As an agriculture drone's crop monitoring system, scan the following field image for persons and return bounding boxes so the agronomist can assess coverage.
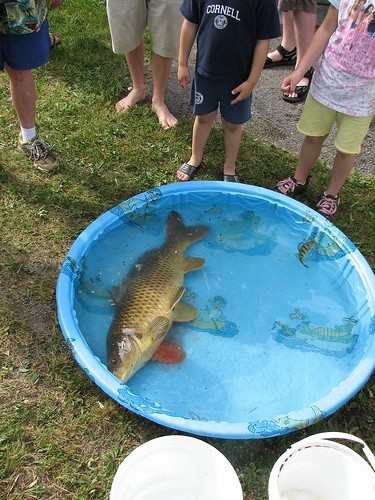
[176,0,281,185]
[270,0,375,219]
[0,0,66,173]
[262,1,319,102]
[106,0,185,131]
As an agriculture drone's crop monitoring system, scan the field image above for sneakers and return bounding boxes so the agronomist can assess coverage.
[273,175,312,197]
[315,191,340,220]
[18,130,59,174]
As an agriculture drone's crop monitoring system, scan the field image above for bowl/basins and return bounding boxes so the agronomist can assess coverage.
[56,180,375,440]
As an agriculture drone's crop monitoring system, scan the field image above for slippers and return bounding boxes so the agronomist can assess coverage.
[222,162,239,183]
[176,157,207,182]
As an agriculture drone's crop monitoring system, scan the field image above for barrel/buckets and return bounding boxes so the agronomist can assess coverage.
[267,431,375,500]
[108,435,243,500]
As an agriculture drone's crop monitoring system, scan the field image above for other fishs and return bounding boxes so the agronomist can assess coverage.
[108,210,211,383]
[298,240,316,268]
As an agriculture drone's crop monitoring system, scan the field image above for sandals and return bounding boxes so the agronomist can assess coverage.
[281,66,315,102]
[263,44,297,69]
